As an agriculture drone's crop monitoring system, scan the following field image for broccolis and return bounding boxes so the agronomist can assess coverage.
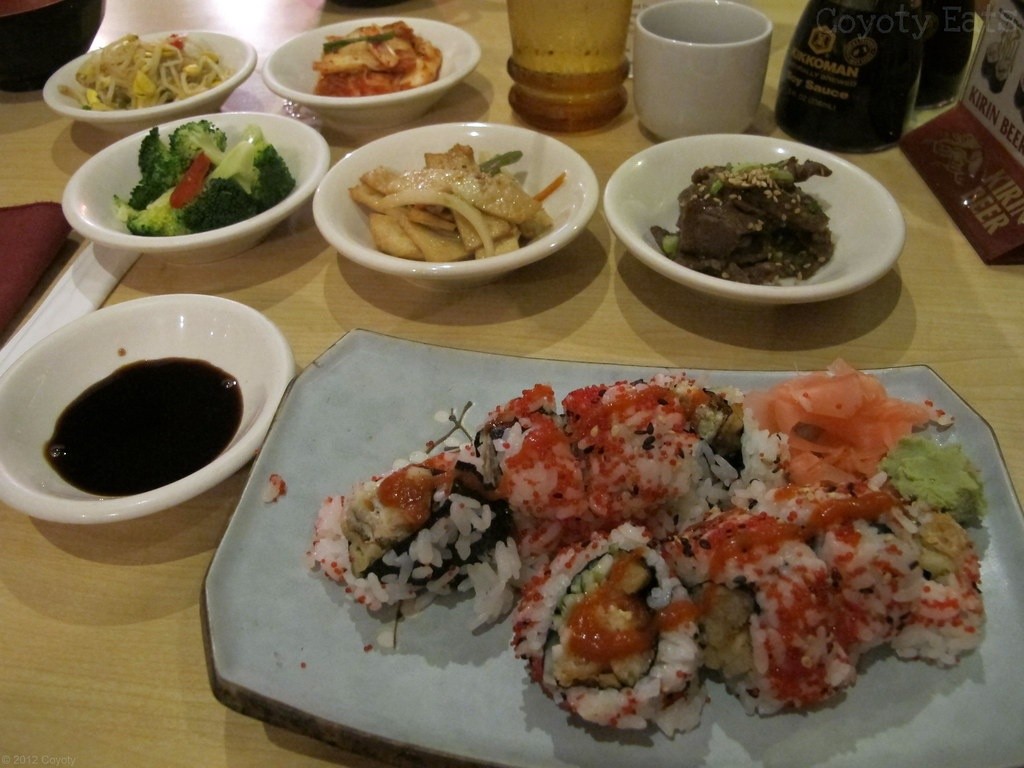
[114,120,294,237]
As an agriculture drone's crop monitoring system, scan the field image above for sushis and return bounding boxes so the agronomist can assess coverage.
[313,371,985,733]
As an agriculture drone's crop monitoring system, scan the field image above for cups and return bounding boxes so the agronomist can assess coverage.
[633,1,773,143]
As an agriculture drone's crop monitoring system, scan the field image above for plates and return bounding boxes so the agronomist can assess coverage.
[604,132,905,304]
[199,328,1024,768]
[42,30,258,136]
[312,121,600,286]
[0,293,301,522]
[260,15,483,128]
[60,111,332,266]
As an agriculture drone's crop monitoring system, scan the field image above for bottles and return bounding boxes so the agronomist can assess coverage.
[776,0,934,154]
[915,0,977,108]
